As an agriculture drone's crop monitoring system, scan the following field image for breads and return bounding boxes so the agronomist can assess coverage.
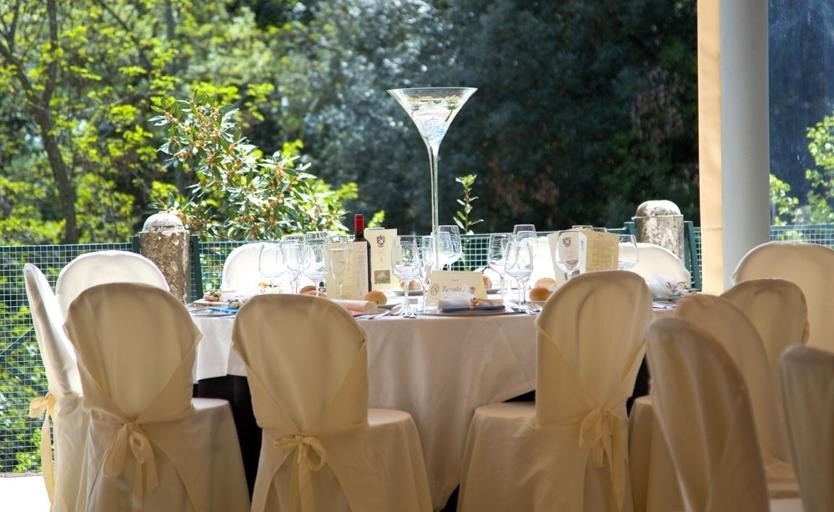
[299,286,316,293]
[408,280,422,291]
[307,290,325,297]
[366,290,388,304]
[400,281,403,286]
[530,287,549,300]
[537,278,556,287]
[481,274,493,289]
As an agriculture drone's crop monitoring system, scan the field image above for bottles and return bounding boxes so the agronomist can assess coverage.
[353,212,373,292]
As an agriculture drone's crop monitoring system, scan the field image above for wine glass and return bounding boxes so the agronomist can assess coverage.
[385,83,480,269]
[278,230,352,315]
[389,223,463,317]
[488,222,641,312]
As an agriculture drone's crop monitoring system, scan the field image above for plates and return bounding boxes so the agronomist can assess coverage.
[190,309,237,318]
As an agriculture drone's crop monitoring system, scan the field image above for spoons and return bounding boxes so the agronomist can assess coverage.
[366,302,404,321]
